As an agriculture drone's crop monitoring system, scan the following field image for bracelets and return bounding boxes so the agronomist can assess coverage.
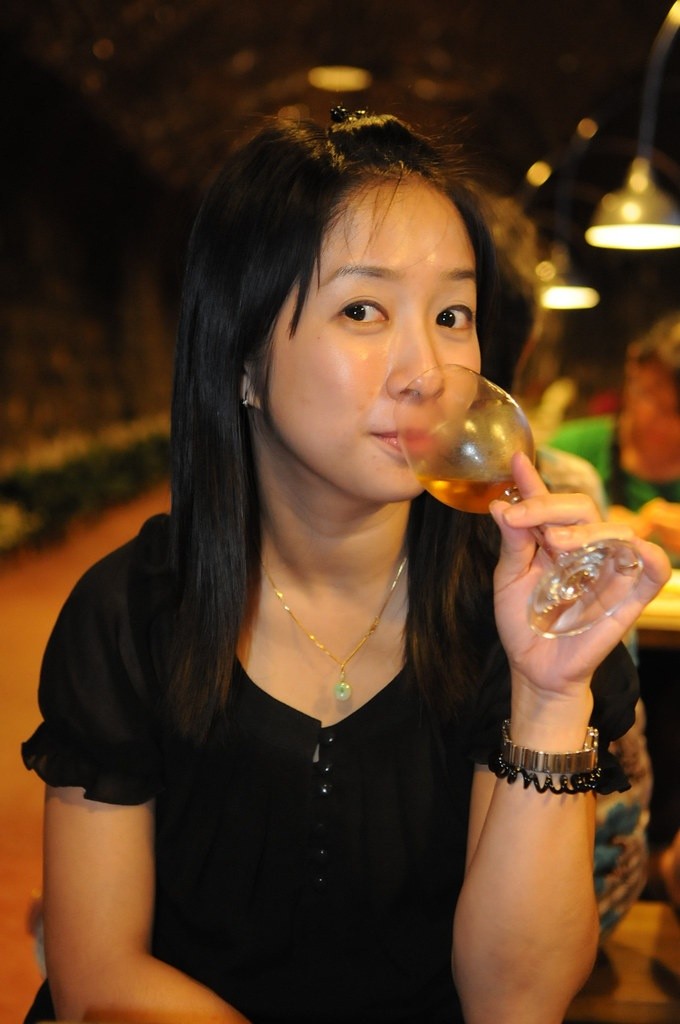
[487,758,604,794]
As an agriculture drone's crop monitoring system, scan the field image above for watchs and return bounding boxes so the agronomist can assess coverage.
[499,720,600,776]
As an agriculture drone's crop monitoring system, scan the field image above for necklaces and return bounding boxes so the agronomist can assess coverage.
[247,542,411,703]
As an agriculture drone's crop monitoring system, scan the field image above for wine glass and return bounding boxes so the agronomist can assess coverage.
[392,365,640,641]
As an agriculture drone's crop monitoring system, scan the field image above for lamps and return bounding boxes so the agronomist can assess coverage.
[586,158,679,249]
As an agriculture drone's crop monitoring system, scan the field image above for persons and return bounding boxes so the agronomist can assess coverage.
[18,100,672,1024]
[540,311,679,910]
